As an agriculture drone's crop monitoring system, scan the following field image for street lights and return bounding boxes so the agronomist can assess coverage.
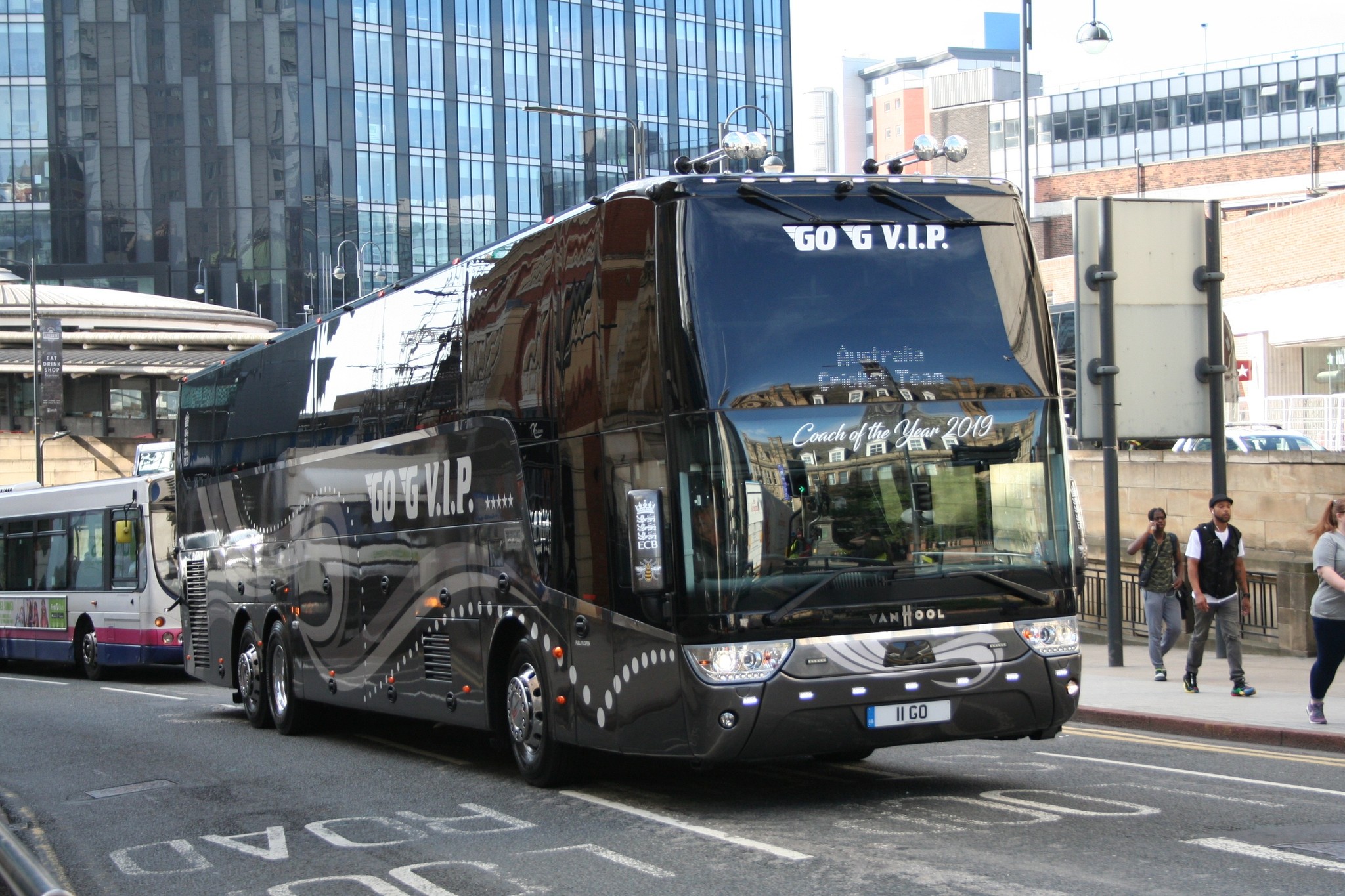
[718,105,787,175]
[1019,0,1113,222]
[36,429,72,486]
[193,258,208,304]
[296,305,315,324]
[331,239,387,300]
[522,106,646,182]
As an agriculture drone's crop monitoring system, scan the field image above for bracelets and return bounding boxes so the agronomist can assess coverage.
[1242,593,1251,598]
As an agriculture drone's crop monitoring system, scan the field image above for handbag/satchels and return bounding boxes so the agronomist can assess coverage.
[1173,581,1192,610]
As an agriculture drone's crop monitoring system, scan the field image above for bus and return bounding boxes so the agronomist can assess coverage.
[0,441,178,682]
[177,106,1085,789]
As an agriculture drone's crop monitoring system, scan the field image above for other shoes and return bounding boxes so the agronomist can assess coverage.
[1154,668,1167,681]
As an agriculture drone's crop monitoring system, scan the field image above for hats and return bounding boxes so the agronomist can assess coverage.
[1208,494,1233,508]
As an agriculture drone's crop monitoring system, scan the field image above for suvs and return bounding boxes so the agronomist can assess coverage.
[1172,422,1329,455]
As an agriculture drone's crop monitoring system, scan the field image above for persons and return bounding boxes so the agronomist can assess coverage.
[1306,500,1345,724]
[1127,507,1184,681]
[691,494,748,579]
[1182,493,1256,697]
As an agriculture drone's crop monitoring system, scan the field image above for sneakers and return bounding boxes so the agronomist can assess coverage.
[1183,673,1199,693]
[1231,678,1256,697]
[1306,699,1327,724]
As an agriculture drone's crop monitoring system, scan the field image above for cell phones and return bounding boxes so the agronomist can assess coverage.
[1150,521,1157,530]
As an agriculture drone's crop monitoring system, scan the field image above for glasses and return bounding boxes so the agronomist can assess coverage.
[1154,516,1166,520]
